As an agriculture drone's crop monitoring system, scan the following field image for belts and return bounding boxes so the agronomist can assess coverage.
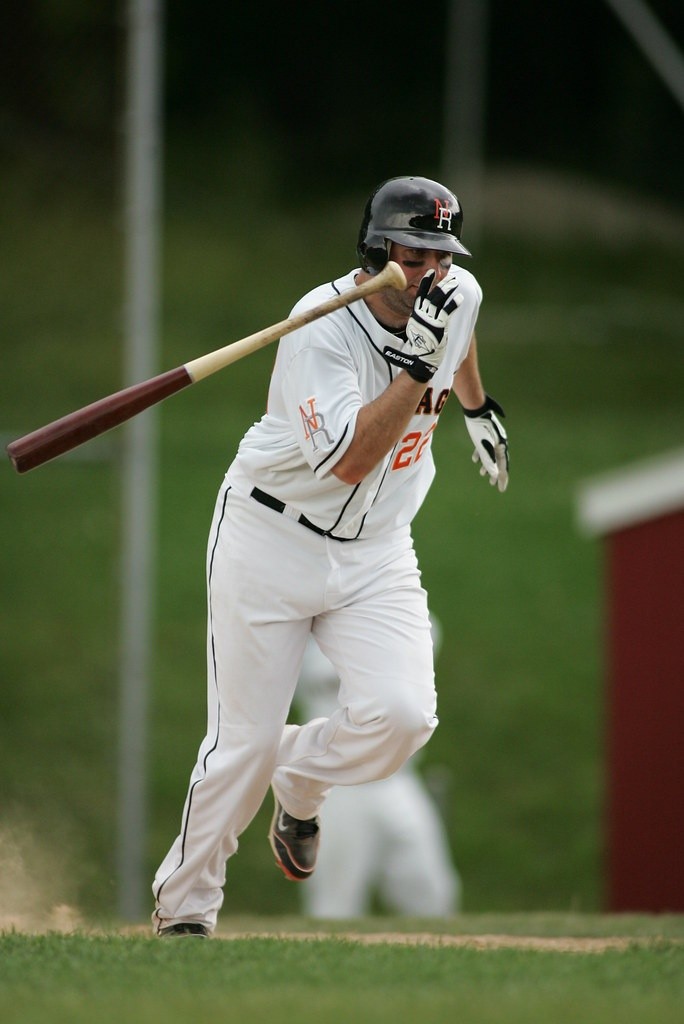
[252,489,326,536]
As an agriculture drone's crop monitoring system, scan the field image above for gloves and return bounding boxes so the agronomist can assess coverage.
[462,395,512,492]
[381,270,464,383]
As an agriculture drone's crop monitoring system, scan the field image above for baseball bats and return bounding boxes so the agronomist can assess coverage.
[6,256,410,475]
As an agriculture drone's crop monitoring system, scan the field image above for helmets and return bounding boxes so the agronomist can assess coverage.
[357,176,472,277]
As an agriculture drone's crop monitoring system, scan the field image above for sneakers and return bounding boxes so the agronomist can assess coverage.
[153,923,214,942]
[269,796,322,879]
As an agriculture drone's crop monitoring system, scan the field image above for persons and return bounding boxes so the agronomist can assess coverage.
[151,174,510,933]
[297,628,458,922]
